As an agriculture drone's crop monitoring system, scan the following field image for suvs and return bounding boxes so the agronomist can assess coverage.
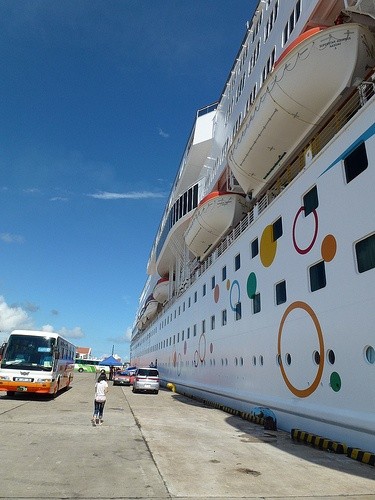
[132,368,160,394]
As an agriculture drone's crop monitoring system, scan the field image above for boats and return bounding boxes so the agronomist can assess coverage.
[228,21,375,204]
[136,300,159,331]
[183,191,246,264]
[152,276,176,306]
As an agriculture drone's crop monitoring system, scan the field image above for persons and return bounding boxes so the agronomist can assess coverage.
[110,368,113,381]
[91,373,108,426]
[149,362,153,367]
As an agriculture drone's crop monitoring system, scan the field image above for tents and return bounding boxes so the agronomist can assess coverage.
[98,355,123,382]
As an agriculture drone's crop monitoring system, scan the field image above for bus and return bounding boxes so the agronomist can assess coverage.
[74,358,110,374]
[0,329,76,400]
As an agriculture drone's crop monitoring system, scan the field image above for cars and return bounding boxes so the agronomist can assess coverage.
[112,370,131,386]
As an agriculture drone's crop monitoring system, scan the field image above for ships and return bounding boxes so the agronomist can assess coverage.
[129,0,375,455]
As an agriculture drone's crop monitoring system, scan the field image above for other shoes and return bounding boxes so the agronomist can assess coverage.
[91,418,96,427]
[98,419,103,424]
